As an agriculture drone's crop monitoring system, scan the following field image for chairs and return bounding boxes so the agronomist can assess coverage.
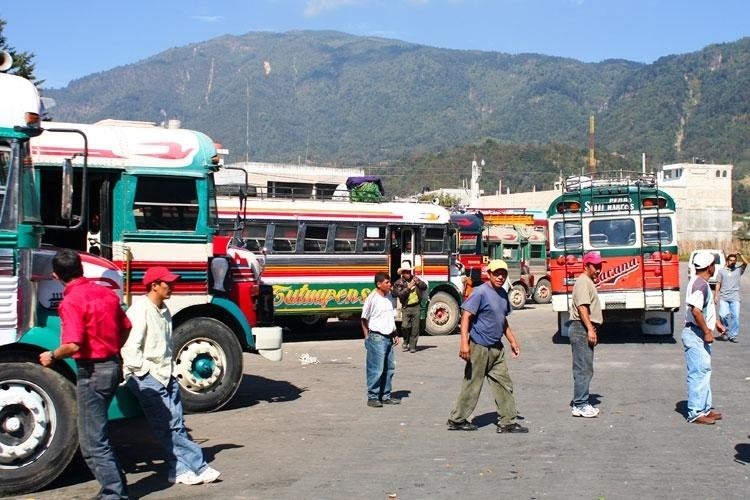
[245,238,389,253]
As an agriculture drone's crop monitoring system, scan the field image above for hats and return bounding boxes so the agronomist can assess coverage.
[583,252,607,265]
[694,253,715,270]
[397,262,416,275]
[143,267,180,286]
[487,259,509,274]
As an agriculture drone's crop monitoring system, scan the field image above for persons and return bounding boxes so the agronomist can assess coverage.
[361,270,400,409]
[119,265,222,487]
[39,248,134,499]
[566,253,608,418]
[445,257,528,433]
[680,250,726,426]
[713,250,748,345]
[393,259,428,353]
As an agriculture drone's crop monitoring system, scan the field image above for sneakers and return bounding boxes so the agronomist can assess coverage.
[497,424,528,433]
[571,405,599,418]
[447,420,478,431]
[708,412,722,420]
[381,398,400,404]
[367,398,383,407]
[695,417,714,425]
[168,467,220,484]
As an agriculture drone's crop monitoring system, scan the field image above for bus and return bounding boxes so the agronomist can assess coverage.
[480,225,534,310]
[448,210,485,288]
[211,185,467,335]
[547,169,680,342]
[0,50,146,497]
[20,118,282,414]
[520,230,552,303]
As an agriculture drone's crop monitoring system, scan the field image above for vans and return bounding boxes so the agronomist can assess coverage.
[688,249,726,288]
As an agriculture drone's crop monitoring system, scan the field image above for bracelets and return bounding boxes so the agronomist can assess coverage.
[705,329,712,336]
[47,350,57,363]
[738,253,742,256]
